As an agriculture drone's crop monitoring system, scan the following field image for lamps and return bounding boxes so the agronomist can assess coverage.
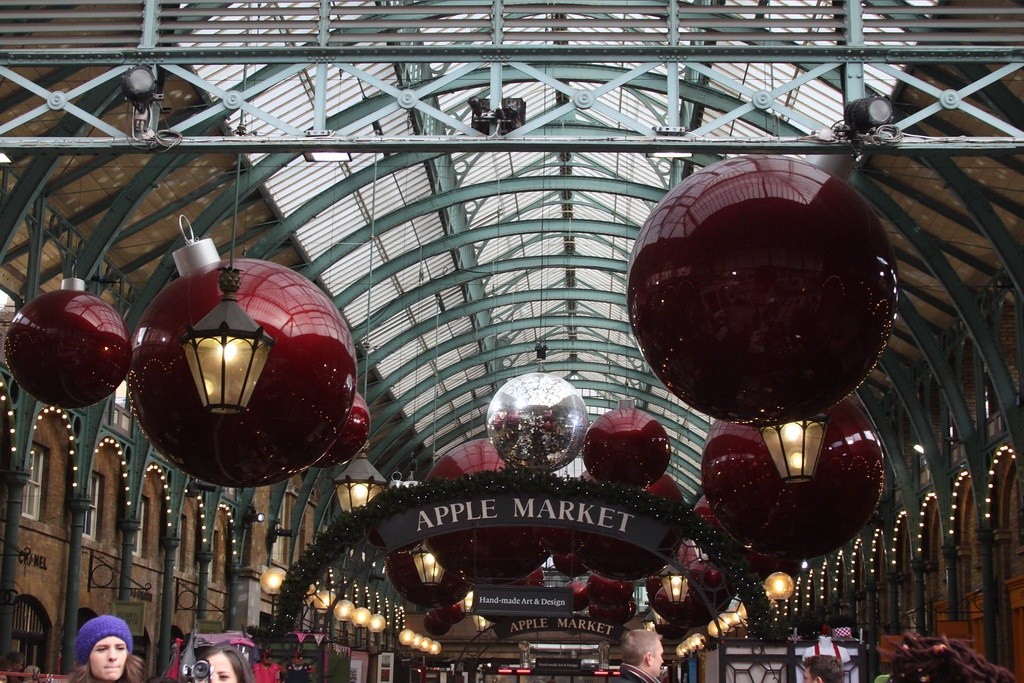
[330,131,391,515]
[243,512,265,522]
[459,590,478,616]
[661,398,690,605]
[259,566,387,634]
[120,66,159,115]
[756,0,838,481]
[275,528,296,539]
[393,629,442,656]
[302,150,352,163]
[641,608,666,639]
[409,293,446,588]
[844,95,894,137]
[472,615,492,632]
[676,633,706,658]
[470,96,528,134]
[707,571,795,638]
[176,3,277,415]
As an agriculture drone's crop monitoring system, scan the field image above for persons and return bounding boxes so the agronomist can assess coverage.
[802,636,851,662]
[193,645,257,683]
[887,631,1018,683]
[804,656,843,683]
[612,629,664,683]
[67,615,145,683]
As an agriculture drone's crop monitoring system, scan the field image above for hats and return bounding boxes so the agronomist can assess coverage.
[74,615,132,666]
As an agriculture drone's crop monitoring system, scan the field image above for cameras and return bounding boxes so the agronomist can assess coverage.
[183,659,211,683]
